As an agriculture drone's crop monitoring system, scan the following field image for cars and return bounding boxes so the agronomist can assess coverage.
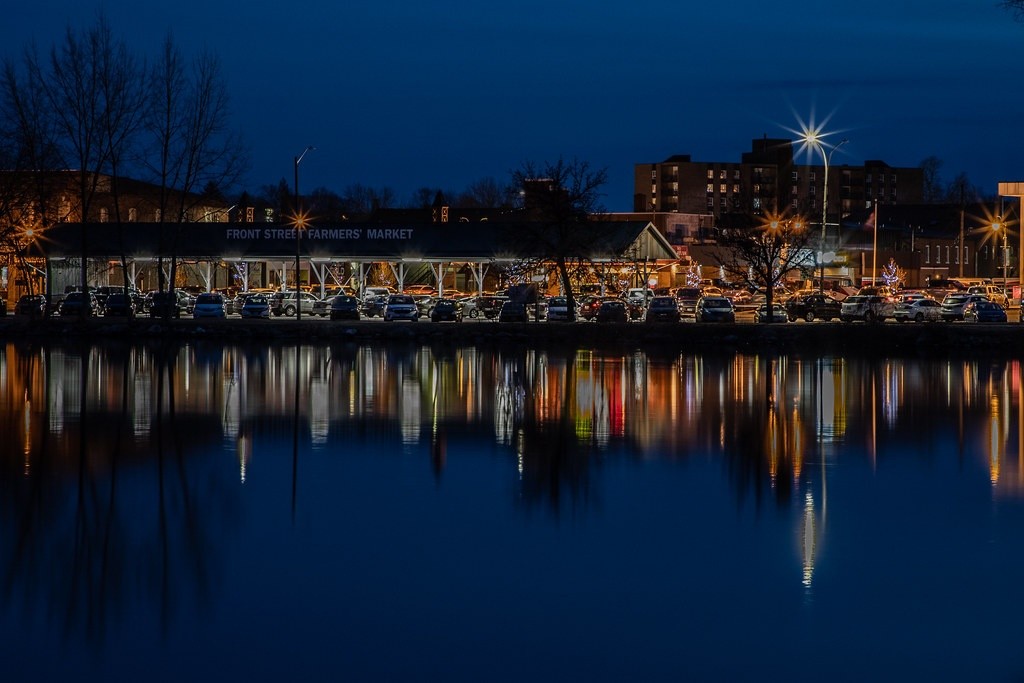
[644,296,683,321]
[695,298,737,323]
[580,297,642,322]
[620,277,857,305]
[963,302,1008,323]
[858,279,967,301]
[893,299,943,322]
[1,283,627,323]
[755,304,789,325]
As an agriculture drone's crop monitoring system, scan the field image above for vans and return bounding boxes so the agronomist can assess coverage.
[948,276,1020,292]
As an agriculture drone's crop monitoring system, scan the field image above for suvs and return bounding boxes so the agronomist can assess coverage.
[787,294,842,322]
[841,295,899,322]
[939,296,990,322]
[966,286,1009,309]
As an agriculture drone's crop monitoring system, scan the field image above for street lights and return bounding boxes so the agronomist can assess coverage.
[992,215,1008,293]
[16,230,33,303]
[805,132,849,292]
[770,215,802,282]
[295,145,318,321]
